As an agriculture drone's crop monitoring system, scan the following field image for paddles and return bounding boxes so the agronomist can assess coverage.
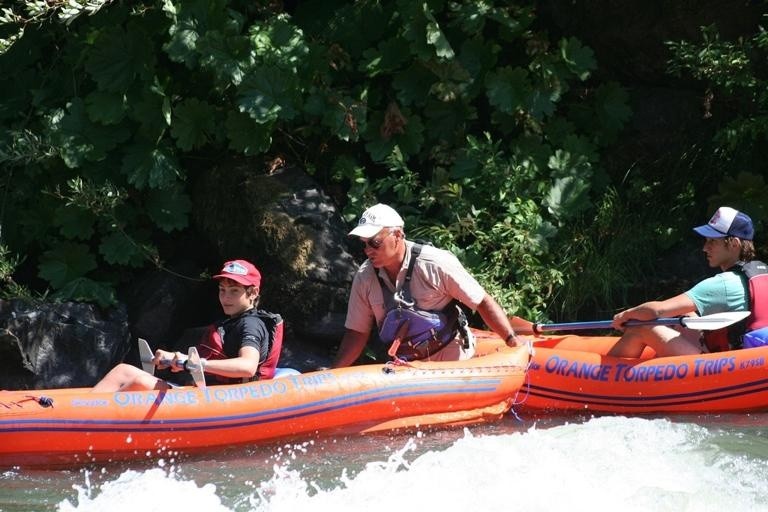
[136,338,207,401]
[508,311,751,331]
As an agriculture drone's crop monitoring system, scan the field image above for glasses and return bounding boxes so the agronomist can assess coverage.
[355,231,392,249]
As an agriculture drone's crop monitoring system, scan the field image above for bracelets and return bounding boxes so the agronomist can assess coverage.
[504,333,517,344]
[199,357,206,372]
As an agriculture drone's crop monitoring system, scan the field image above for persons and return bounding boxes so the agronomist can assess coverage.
[85,259,270,394]
[300,203,525,375]
[606,207,768,359]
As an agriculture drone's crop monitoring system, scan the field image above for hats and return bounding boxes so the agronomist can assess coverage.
[347,203,405,239]
[692,206,755,240]
[212,259,262,287]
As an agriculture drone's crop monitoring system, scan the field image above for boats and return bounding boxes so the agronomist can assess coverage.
[430,327,768,413]
[0,338,531,469]
